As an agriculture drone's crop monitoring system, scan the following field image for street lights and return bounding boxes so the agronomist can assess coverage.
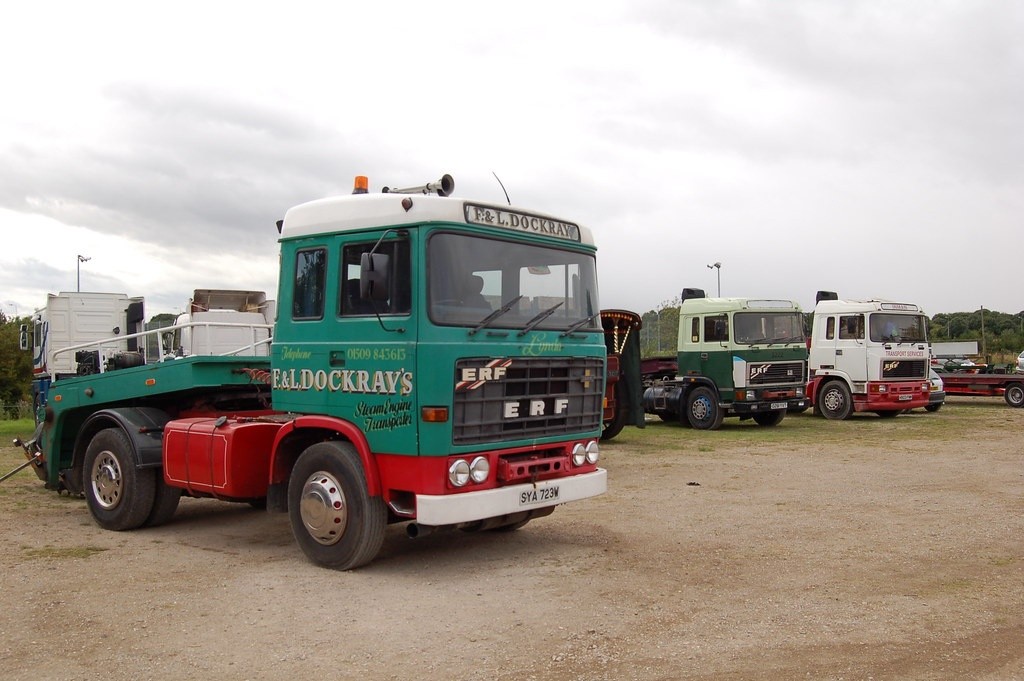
[707,262,722,297]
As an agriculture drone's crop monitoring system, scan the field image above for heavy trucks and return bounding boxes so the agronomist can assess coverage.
[36,175,608,572]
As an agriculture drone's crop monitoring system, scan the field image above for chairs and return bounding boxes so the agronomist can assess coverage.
[347,278,389,313]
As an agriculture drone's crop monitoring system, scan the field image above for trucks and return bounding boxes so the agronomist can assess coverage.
[21,291,144,429]
[643,288,811,430]
[786,291,934,422]
[162,312,270,356]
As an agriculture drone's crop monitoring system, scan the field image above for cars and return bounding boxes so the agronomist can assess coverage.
[900,368,945,415]
[1016,351,1024,374]
